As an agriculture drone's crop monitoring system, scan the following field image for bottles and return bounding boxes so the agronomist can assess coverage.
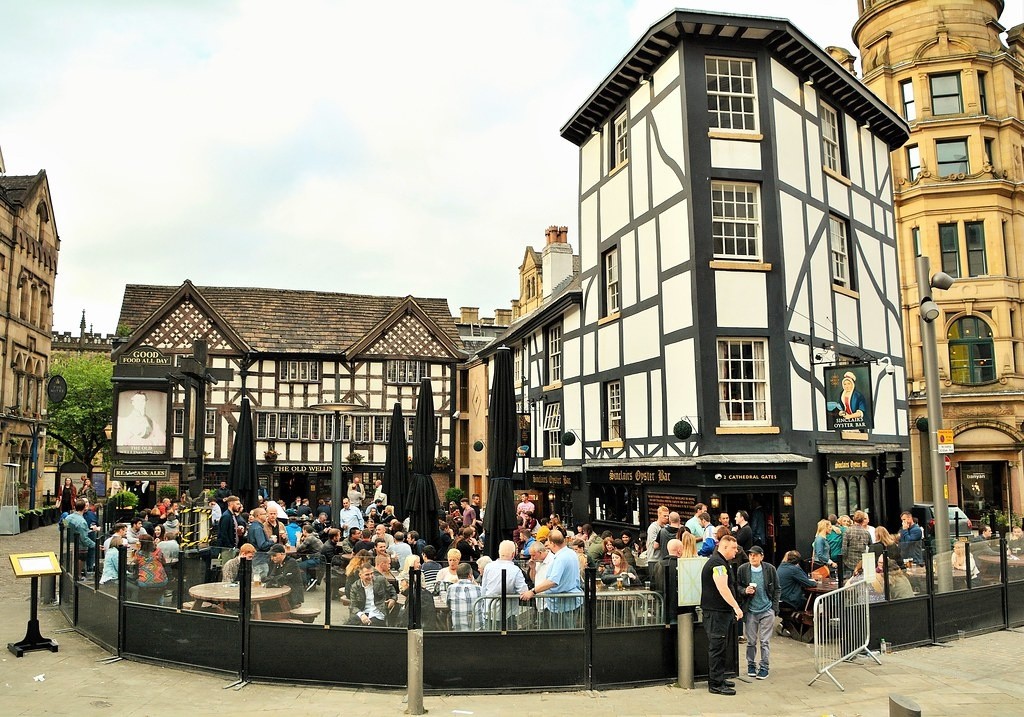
[880,638,886,655]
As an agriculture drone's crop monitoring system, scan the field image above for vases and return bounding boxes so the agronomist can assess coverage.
[436,463,447,468]
[351,460,360,464]
[266,457,276,462]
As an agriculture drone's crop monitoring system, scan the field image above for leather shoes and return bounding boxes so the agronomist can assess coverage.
[724,679,736,687]
[710,684,736,695]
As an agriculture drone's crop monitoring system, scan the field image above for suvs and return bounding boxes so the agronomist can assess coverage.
[914,504,975,539]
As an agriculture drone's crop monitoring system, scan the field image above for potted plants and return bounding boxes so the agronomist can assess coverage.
[998,516,1007,538]
[19,505,60,533]
[158,484,177,503]
[111,493,139,523]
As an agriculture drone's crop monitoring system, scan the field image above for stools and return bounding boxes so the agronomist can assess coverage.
[183,601,212,613]
[338,586,352,605]
[290,607,322,624]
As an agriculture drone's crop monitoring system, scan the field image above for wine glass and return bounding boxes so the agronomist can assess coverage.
[919,560,925,574]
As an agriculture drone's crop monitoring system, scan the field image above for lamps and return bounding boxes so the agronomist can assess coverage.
[548,491,554,501]
[674,415,703,440]
[473,439,488,452]
[783,491,793,510]
[907,414,929,433]
[561,429,582,447]
[710,493,720,513]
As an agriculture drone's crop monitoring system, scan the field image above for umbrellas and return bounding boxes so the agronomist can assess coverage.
[229,396,259,513]
[484,344,518,560]
[408,375,440,548]
[382,401,409,522]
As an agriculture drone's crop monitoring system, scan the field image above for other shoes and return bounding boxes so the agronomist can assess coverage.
[784,629,794,639]
[801,626,814,642]
[789,626,802,642]
[775,622,784,633]
[306,577,317,591]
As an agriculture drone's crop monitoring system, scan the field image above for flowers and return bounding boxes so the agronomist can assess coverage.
[434,456,449,469]
[264,450,282,460]
[346,453,365,464]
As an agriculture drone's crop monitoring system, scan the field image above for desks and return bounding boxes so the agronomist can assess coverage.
[284,545,297,554]
[342,554,353,560]
[596,585,655,601]
[189,582,293,620]
[805,583,839,612]
[395,594,452,631]
[906,567,967,579]
[336,541,343,546]
[978,553,1024,568]
[387,575,397,581]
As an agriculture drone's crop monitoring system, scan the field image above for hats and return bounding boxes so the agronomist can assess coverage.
[267,544,285,555]
[747,545,764,554]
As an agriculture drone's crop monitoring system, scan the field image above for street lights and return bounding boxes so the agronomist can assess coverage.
[916,255,957,592]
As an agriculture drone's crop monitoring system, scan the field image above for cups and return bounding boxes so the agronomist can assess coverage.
[279,529,285,537]
[136,542,140,549]
[616,578,623,592]
[886,642,892,654]
[808,572,813,581]
[253,575,267,593]
[644,581,650,590]
[455,509,460,517]
[903,558,917,574]
[271,535,277,540]
[817,575,822,584]
[835,570,839,582]
[749,583,758,596]
[237,526,242,536]
[285,544,291,551]
[596,577,602,589]
[951,563,956,574]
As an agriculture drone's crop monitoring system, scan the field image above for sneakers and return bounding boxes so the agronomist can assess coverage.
[756,669,768,680]
[748,664,757,677]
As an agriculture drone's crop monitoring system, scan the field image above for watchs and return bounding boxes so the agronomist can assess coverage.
[531,588,537,595]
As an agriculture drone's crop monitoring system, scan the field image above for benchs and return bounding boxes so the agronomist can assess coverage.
[776,602,814,643]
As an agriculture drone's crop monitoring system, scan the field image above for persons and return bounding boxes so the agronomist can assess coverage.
[66,476,752,631]
[775,510,924,638]
[951,540,980,588]
[737,545,781,680]
[974,497,986,517]
[55,478,78,515]
[1006,526,1024,571]
[700,535,745,695]
[347,477,366,500]
[972,524,1011,569]
